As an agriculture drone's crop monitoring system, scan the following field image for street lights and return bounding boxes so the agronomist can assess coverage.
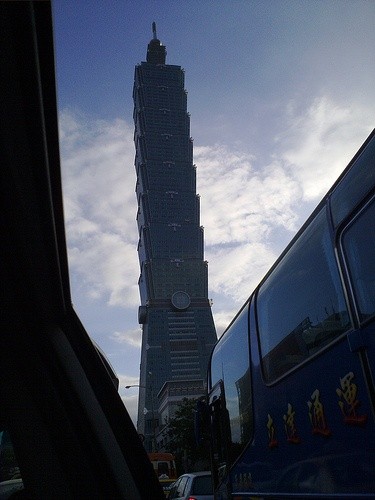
[124,384,158,452]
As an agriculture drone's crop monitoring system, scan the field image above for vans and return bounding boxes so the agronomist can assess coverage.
[167,471,215,500]
[147,452,178,481]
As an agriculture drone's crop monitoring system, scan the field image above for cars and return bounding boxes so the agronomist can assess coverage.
[158,473,179,499]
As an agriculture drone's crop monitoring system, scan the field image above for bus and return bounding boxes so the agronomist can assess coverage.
[191,126,375,500]
